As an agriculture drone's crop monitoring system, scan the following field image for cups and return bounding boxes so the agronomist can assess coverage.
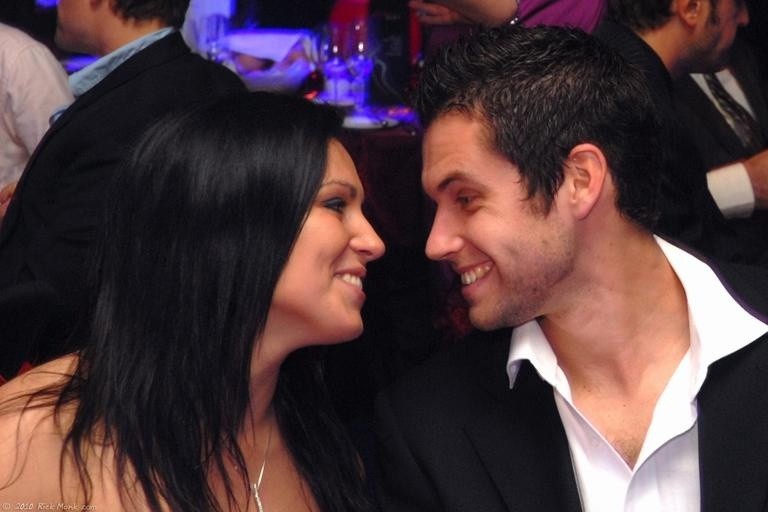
[202,12,231,66]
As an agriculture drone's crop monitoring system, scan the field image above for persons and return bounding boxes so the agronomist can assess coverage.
[0,22,76,219]
[407,1,603,36]
[370,20,767,511]
[587,2,768,268]
[1,1,250,384]
[0,90,391,512]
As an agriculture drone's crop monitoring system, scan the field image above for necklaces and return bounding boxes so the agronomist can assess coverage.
[221,425,273,512]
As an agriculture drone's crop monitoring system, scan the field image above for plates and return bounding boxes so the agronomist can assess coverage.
[342,115,399,130]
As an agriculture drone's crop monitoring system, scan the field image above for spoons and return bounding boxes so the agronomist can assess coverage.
[374,115,388,127]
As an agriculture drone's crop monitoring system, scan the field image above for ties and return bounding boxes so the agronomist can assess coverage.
[704,71,761,146]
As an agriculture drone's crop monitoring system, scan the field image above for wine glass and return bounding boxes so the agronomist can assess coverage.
[319,22,350,111]
[346,19,378,112]
